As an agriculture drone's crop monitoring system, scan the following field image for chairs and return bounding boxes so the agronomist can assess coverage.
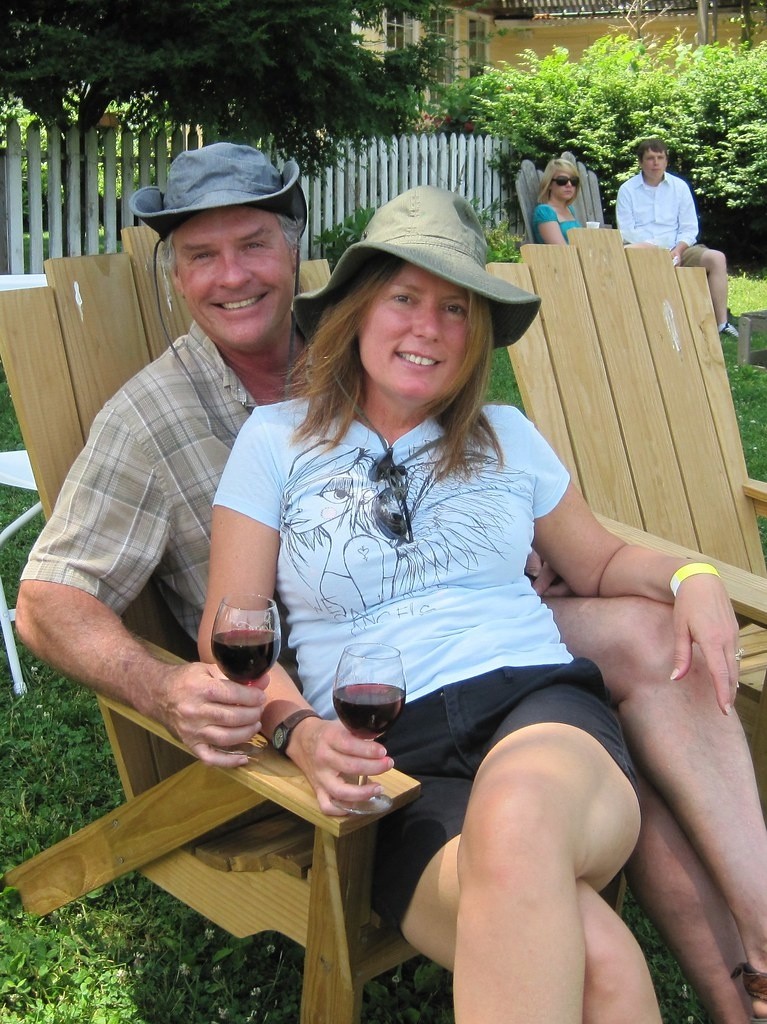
[560,150,604,227]
[484,228,766,831]
[0,223,628,1024]
[0,272,53,694]
[515,159,545,244]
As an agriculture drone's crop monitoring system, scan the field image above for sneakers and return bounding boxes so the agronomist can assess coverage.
[719,322,738,339]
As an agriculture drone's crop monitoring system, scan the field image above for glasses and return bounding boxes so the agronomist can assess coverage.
[366,449,413,546]
[550,176,580,188]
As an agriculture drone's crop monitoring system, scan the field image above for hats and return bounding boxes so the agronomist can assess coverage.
[127,143,309,239]
[292,185,540,380]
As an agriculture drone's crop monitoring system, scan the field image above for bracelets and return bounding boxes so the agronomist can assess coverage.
[669,562,721,596]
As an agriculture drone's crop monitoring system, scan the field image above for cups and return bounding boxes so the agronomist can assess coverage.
[586,222,600,229]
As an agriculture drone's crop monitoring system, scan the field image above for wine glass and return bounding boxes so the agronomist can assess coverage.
[208,593,281,755]
[331,642,406,815]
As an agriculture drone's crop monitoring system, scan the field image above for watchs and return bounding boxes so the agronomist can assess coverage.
[271,709,321,755]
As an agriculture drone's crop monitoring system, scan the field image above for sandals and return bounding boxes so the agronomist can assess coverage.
[730,962,767,1024]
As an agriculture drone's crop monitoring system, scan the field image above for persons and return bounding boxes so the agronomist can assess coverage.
[616,136,739,337]
[530,158,584,247]
[14,141,766,1024]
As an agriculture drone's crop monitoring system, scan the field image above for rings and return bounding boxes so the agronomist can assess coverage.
[734,648,745,661]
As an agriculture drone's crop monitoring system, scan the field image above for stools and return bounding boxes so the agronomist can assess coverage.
[738,310,767,370]
